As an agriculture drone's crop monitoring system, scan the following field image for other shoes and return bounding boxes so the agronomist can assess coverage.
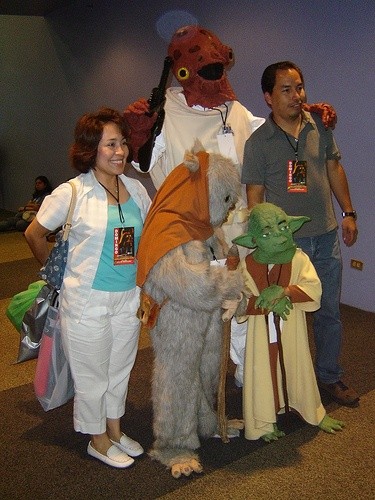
[318,375,360,405]
[87,433,144,468]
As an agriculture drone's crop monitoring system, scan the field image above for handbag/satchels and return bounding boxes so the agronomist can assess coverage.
[39,182,77,290]
[22,200,37,222]
[7,281,75,412]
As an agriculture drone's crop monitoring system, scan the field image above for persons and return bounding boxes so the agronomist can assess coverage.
[0,175,53,231]
[234,202,345,443]
[240,60,358,406]
[24,107,152,469]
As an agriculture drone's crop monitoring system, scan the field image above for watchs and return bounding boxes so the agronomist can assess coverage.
[342,210,356,218]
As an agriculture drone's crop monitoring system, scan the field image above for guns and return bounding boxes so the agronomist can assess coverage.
[138,55,173,172]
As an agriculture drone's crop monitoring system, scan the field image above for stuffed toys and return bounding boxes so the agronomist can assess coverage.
[122,25,338,388]
[136,136,254,479]
